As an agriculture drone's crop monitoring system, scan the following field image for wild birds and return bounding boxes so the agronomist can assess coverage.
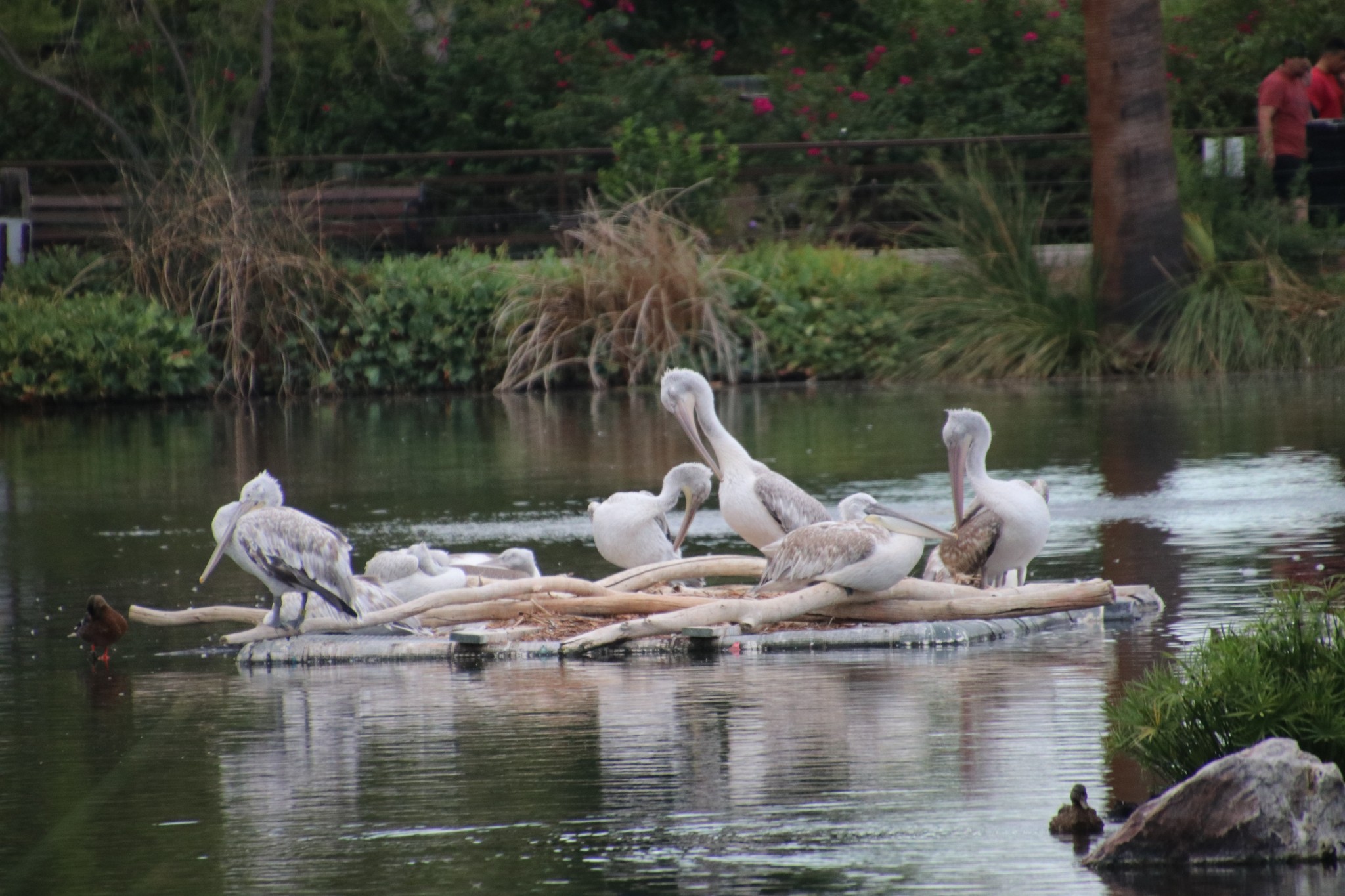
[200,471,360,633]
[263,541,545,639]
[759,490,959,596]
[662,365,827,557]
[590,462,715,572]
[76,592,127,657]
[925,408,1052,589]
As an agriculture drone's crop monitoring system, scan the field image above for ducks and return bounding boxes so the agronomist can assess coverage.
[1046,782,1106,834]
